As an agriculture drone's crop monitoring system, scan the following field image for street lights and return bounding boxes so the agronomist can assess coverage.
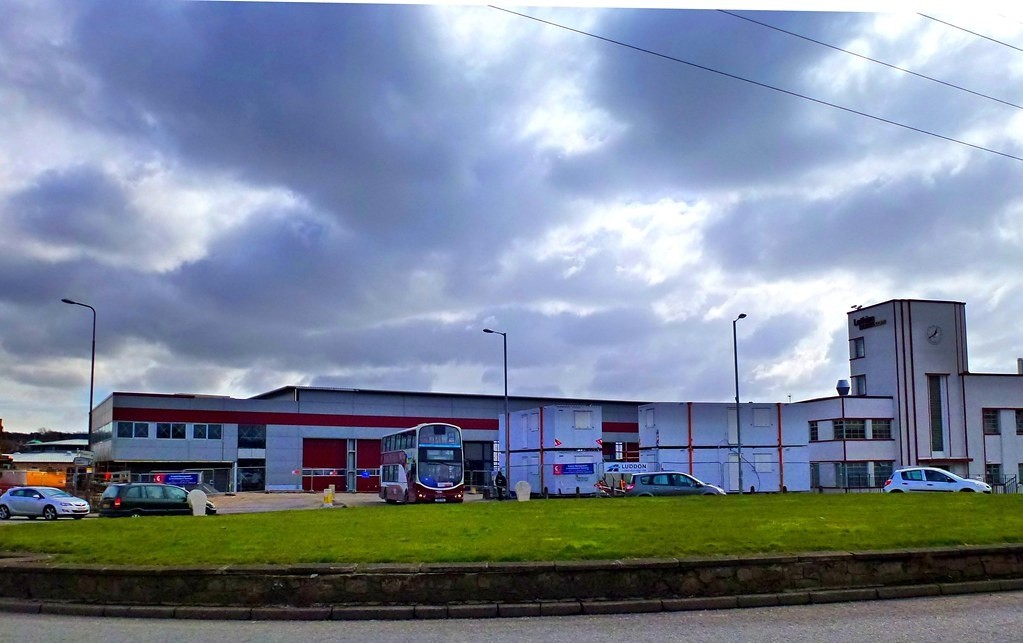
[732,313,748,495]
[483,328,512,500]
[59,297,96,449]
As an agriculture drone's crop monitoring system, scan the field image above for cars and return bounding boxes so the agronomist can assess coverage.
[0,485,90,521]
[883,466,992,494]
[627,471,727,497]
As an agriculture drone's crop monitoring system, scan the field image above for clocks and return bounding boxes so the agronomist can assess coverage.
[927,325,943,344]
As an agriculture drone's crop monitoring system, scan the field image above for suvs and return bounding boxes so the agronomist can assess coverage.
[98,481,218,518]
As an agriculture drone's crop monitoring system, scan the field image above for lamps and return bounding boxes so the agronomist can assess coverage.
[851,304,862,309]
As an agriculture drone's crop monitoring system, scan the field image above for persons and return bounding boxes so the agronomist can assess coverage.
[495,471,507,501]
[977,475,983,481]
[85,489,91,499]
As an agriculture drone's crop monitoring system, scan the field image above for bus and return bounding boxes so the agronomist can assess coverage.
[377,422,465,504]
